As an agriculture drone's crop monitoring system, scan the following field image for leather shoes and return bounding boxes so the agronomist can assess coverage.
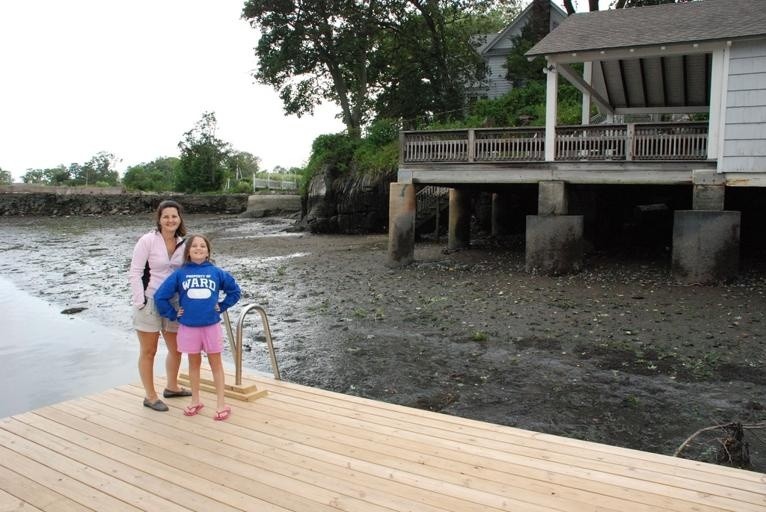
[163,387,192,398]
[142,398,170,412]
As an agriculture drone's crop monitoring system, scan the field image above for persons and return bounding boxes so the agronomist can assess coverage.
[152,233,241,423]
[127,200,192,415]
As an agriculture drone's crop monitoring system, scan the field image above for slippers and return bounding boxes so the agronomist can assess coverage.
[213,406,232,422]
[182,402,204,417]
